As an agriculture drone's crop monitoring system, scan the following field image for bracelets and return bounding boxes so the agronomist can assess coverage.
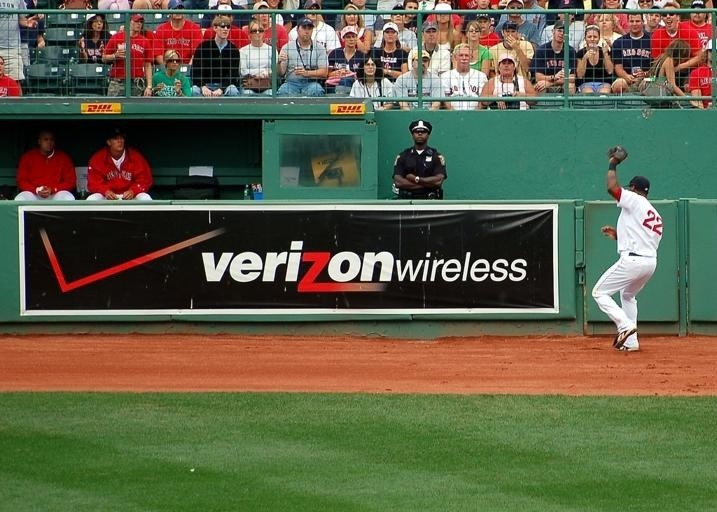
[608,163,616,171]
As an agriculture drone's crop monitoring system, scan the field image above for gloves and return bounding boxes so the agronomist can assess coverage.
[607,144,629,164]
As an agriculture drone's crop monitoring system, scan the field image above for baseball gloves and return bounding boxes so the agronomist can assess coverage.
[607,146,628,162]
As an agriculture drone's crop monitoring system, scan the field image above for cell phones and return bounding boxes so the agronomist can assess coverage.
[590,43,597,53]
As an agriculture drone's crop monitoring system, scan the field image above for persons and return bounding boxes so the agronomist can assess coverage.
[392,120,447,199]
[0,0,716,112]
[85,127,153,201]
[592,146,663,352]
[15,130,77,201]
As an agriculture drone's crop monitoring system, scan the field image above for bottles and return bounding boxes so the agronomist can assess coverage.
[80,186,86,198]
[243,184,249,201]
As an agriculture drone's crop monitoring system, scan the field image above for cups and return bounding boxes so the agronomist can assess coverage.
[294,67,302,77]
[117,44,124,58]
[588,43,596,54]
[630,66,640,78]
[338,64,346,78]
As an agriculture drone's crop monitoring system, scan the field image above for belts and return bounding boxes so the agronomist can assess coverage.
[628,252,643,256]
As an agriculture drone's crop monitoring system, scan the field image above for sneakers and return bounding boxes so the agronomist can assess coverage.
[612,326,640,352]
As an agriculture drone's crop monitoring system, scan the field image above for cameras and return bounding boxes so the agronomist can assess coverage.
[304,64,317,70]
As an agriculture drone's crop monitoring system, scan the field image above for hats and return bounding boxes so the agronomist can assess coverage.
[408,119,433,136]
[107,126,125,140]
[622,175,651,194]
[125,2,706,64]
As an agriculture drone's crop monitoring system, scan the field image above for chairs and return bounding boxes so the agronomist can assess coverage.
[22,11,207,98]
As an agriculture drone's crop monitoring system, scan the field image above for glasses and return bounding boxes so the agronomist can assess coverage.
[413,129,428,133]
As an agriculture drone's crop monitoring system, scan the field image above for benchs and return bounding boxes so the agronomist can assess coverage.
[1,166,260,200]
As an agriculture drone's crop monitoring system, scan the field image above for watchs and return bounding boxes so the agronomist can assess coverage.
[415,176,419,184]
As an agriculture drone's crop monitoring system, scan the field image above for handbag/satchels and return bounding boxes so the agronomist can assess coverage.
[242,74,272,89]
[638,54,675,108]
